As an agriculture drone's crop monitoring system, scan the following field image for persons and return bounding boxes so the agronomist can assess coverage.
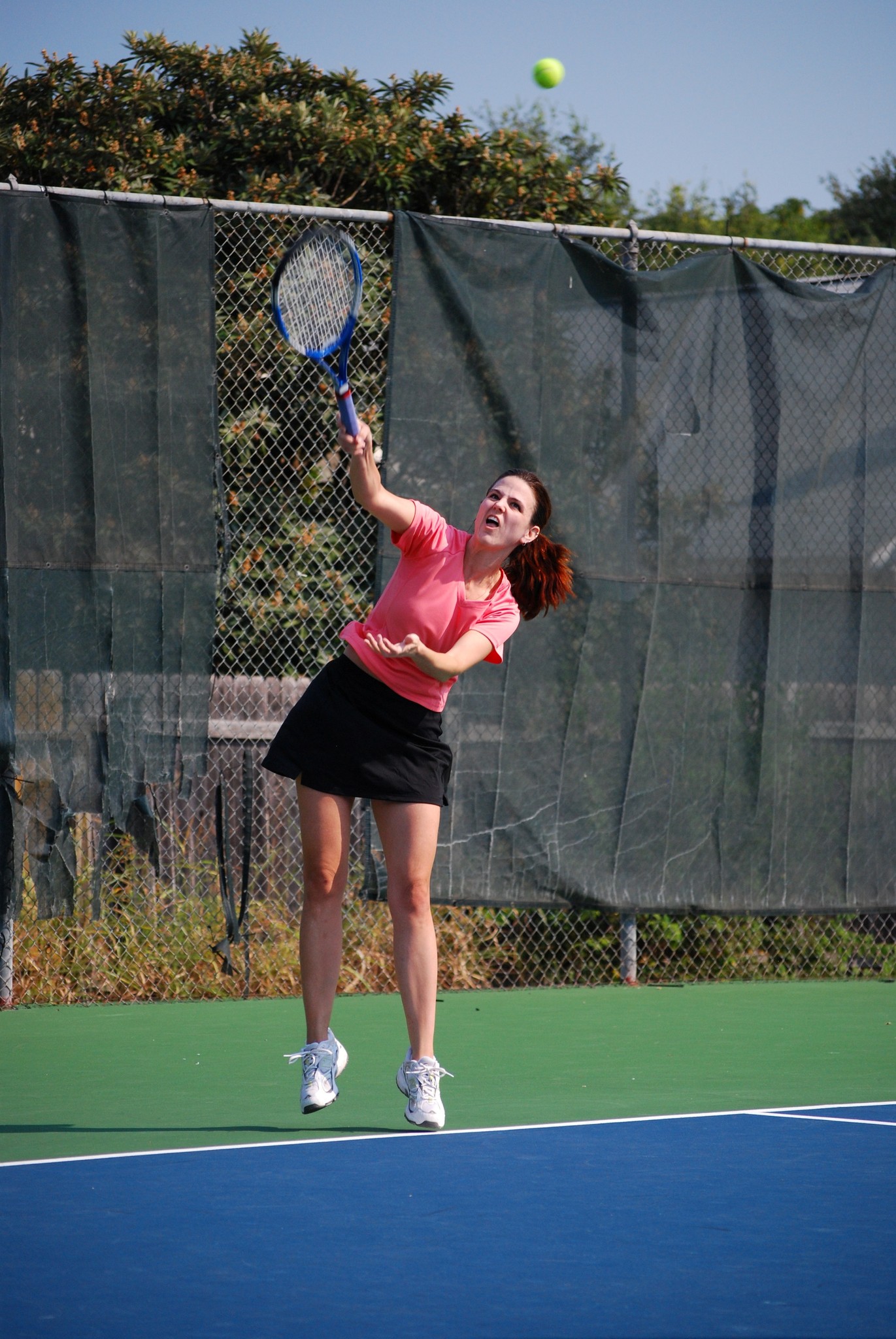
[259,419,574,1131]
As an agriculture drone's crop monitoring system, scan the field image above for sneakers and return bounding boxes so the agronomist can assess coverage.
[283,1027,348,1114]
[395,1045,454,1129]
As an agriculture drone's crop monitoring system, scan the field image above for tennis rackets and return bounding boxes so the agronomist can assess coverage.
[271,224,363,436]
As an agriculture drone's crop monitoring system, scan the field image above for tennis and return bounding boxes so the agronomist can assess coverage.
[533,57,566,89]
